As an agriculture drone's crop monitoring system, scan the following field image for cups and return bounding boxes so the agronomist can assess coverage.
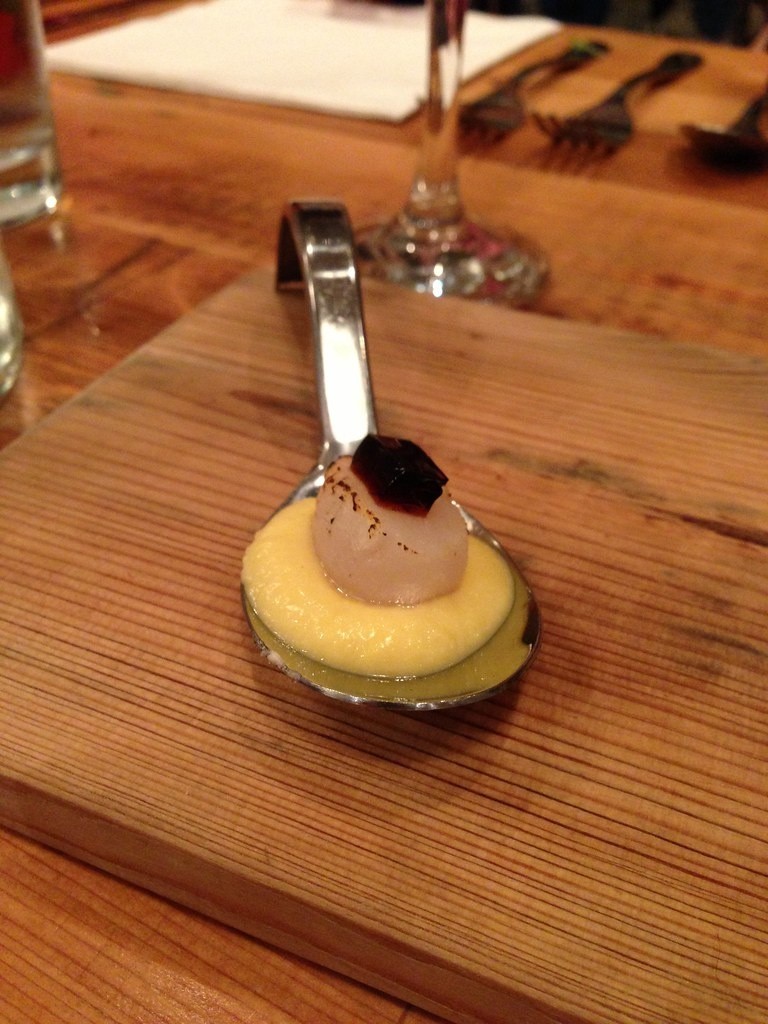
[0,0,62,230]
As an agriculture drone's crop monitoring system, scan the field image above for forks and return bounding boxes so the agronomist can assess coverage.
[455,39,609,147]
[534,52,703,152]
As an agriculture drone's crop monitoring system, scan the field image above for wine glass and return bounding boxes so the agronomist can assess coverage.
[351,2,545,305]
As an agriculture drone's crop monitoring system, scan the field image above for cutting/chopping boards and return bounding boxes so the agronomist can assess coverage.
[0,248,768,1024]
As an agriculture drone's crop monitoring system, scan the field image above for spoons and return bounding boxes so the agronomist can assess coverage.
[241,197,542,712]
[683,87,768,169]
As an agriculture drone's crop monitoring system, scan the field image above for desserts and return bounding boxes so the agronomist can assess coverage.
[241,432,536,703]
